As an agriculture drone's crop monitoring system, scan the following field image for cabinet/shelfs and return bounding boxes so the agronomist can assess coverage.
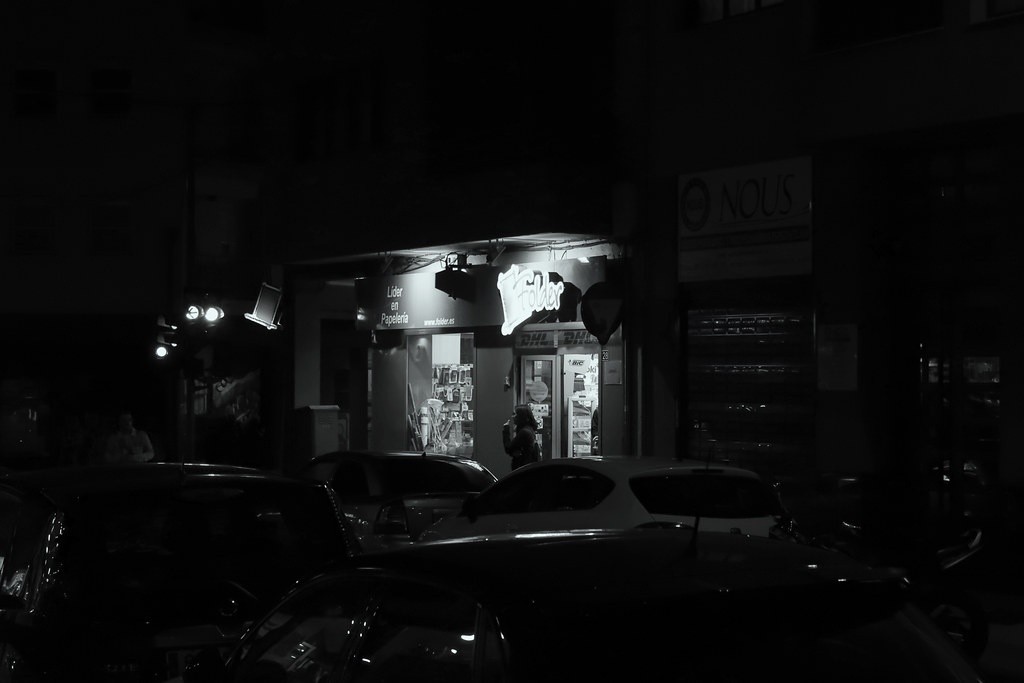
[567,397,597,458]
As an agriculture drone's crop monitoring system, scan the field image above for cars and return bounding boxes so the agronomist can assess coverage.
[418,455,792,541]
[181,527,993,683]
[0,457,372,682]
[297,449,500,556]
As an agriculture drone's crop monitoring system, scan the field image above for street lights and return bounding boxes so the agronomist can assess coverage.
[147,293,229,464]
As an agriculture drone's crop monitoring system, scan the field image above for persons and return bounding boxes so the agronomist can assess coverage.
[591,407,599,456]
[56,409,155,465]
[206,418,274,467]
[502,405,540,471]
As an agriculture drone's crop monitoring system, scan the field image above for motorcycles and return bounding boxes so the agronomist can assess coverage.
[770,472,992,666]
